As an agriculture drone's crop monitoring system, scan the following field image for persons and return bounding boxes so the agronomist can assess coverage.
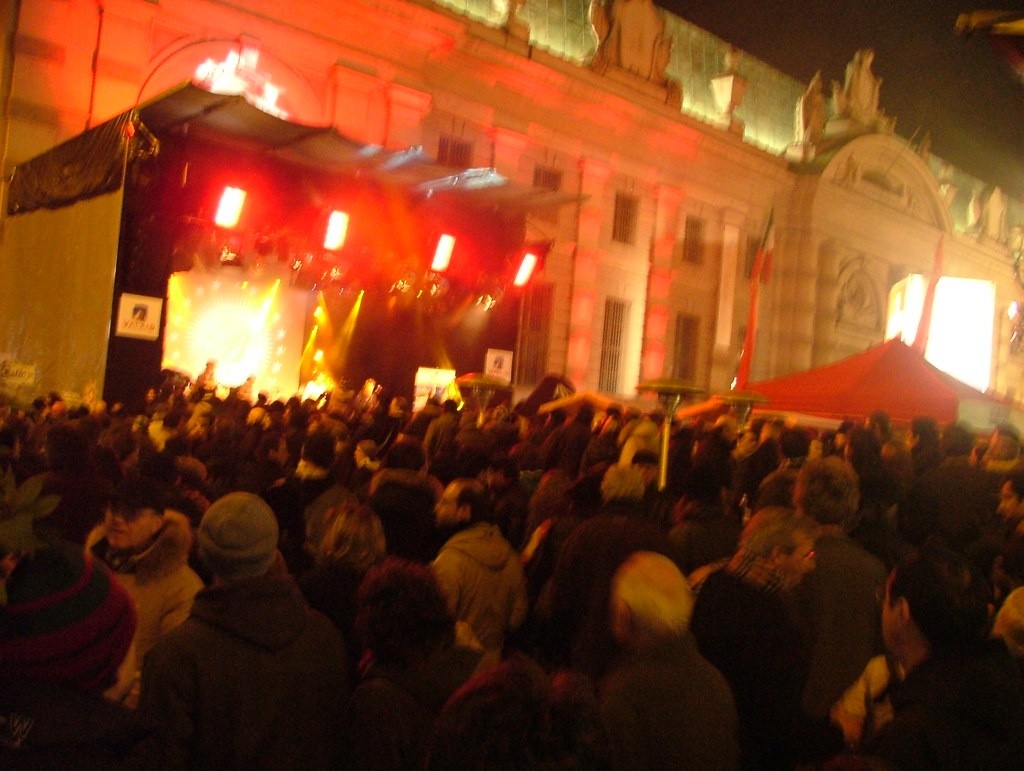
[0,362,1024,771]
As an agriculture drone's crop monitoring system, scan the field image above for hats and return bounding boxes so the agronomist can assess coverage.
[101,482,159,511]
[197,492,279,574]
[0,540,139,690]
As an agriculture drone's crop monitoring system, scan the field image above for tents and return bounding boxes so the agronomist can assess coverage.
[740,336,1024,423]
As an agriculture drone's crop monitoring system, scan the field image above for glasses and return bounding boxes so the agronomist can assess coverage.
[876,586,897,602]
